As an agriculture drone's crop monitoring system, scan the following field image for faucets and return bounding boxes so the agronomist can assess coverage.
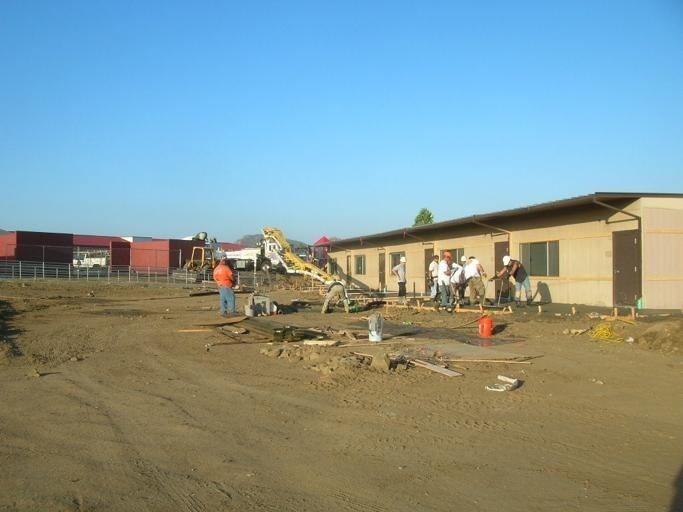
[249,293,254,297]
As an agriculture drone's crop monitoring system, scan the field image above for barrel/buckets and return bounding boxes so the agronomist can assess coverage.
[479,317,493,336]
[245,306,255,316]
[369,320,383,341]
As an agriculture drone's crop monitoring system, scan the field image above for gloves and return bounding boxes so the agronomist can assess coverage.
[491,275,497,279]
[505,273,510,279]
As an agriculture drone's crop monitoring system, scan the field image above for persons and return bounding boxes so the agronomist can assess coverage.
[434,250,454,308]
[211,257,237,317]
[427,254,441,302]
[448,262,465,307]
[391,256,408,298]
[320,279,350,315]
[461,256,488,314]
[491,256,534,307]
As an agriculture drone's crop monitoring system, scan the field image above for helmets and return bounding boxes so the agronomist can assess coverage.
[399,257,406,262]
[502,256,510,266]
[443,251,452,257]
[460,256,466,261]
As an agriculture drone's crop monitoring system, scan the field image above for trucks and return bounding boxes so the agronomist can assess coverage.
[214,241,283,272]
[72,252,105,268]
